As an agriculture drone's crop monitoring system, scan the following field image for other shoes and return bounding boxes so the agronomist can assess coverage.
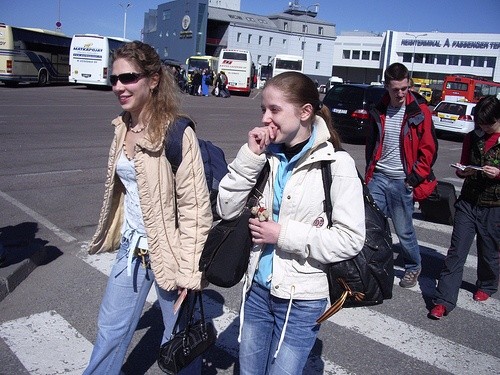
[430,303,446,319]
[473,286,488,301]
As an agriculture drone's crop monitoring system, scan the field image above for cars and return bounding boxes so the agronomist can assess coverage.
[431,102,477,135]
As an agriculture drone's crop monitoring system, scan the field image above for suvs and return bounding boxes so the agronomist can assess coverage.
[321,83,392,145]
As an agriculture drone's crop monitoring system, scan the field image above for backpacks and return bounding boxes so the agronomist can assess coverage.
[164,115,228,230]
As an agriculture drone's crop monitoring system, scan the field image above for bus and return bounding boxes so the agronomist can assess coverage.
[440,76,500,104]
[185,56,220,85]
[326,76,344,92]
[68,34,133,91]
[0,22,73,87]
[218,49,256,96]
[256,54,304,89]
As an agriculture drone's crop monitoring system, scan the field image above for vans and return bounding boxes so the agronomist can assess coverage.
[418,87,432,105]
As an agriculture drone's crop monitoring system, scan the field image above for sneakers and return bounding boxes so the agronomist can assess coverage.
[399,267,422,288]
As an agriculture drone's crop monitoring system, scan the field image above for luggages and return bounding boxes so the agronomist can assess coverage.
[219,89,228,98]
[418,180,458,225]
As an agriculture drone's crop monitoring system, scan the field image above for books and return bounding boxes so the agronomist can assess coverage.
[451,165,484,174]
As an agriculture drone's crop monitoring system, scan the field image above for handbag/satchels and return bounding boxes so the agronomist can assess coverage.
[315,146,394,325]
[157,289,218,375]
[197,158,271,289]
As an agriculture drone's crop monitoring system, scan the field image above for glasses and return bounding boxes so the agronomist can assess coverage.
[109,72,145,86]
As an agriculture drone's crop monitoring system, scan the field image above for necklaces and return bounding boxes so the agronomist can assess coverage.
[124,140,135,160]
[129,119,146,133]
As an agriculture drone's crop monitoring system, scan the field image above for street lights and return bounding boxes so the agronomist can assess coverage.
[120,3,131,38]
[405,32,427,79]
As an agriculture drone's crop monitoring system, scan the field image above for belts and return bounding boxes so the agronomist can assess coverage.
[132,248,150,258]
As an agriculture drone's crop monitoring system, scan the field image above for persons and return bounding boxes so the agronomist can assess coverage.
[172,65,189,93]
[216,71,366,375]
[83,41,214,375]
[215,71,230,97]
[428,96,500,319]
[191,68,210,97]
[365,62,439,288]
